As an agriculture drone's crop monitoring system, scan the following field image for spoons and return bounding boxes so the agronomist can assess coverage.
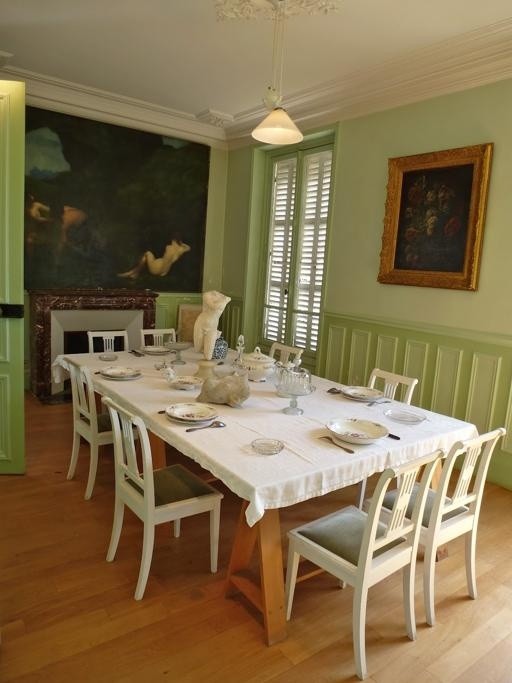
[182,419,227,432]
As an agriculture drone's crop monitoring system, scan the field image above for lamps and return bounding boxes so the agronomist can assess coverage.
[249,1,303,146]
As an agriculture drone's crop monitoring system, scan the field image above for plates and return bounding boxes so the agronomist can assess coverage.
[166,400,221,424]
[340,385,386,400]
[99,353,119,362]
[141,343,171,355]
[383,406,428,425]
[99,365,144,381]
[326,417,389,446]
[250,436,284,455]
[169,375,204,390]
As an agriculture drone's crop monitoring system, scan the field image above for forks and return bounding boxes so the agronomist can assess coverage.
[318,434,355,456]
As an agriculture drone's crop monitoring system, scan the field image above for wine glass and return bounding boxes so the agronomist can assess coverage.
[278,365,310,416]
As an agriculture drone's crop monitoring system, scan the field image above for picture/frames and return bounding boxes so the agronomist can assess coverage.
[375,141,494,291]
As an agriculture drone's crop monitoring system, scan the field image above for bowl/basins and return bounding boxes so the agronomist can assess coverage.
[244,346,283,383]
[171,363,200,376]
